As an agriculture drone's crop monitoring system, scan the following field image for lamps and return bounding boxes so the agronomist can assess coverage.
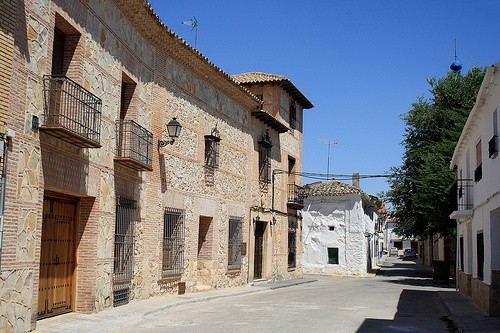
[253,213,260,224]
[157,116,182,150]
[270,216,277,226]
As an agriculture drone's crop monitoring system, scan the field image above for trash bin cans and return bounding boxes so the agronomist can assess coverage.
[432,260,451,288]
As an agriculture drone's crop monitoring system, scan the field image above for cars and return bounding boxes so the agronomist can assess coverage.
[403,248,417,259]
[391,247,397,255]
